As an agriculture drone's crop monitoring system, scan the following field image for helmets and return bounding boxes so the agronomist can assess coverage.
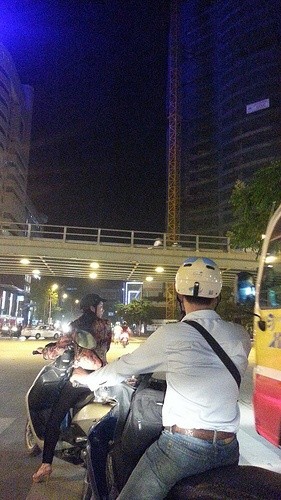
[80,294,107,309]
[175,257,223,299]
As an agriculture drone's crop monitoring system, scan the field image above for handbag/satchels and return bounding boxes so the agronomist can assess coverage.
[120,388,166,456]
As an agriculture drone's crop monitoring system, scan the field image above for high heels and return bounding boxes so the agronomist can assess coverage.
[33,464,52,484]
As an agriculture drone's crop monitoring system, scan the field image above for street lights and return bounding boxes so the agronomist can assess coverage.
[47,283,79,332]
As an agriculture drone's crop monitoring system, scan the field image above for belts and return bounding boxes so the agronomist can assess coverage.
[165,425,234,440]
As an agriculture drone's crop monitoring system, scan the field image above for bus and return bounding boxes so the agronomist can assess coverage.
[231,204,281,448]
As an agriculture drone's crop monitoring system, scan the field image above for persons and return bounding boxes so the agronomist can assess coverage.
[69,256,251,500]
[114,321,123,344]
[33,294,111,487]
[122,321,131,335]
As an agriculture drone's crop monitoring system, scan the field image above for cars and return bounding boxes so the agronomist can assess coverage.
[21,325,60,339]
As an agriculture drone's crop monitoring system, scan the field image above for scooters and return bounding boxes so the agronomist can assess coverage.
[119,332,129,348]
[70,367,281,500]
[25,342,117,465]
